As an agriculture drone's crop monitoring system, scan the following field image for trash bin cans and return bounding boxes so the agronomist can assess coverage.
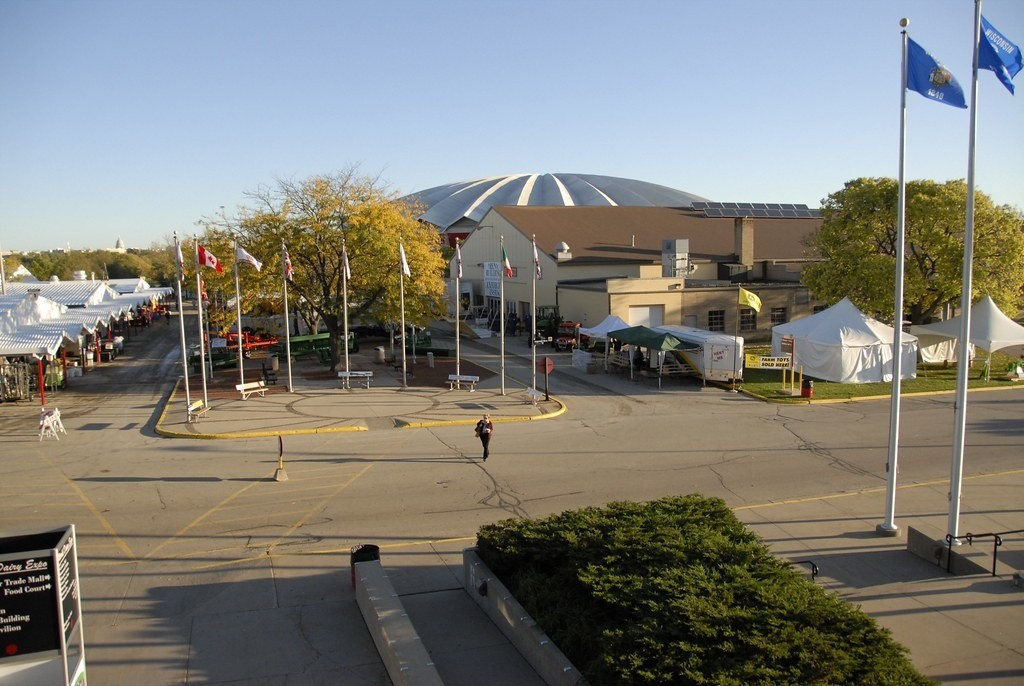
[373,345,386,365]
[271,355,279,371]
[802,380,814,399]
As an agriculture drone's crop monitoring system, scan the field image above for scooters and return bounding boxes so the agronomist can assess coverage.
[43,364,65,391]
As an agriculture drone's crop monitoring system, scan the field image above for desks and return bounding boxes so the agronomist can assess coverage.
[589,352,605,368]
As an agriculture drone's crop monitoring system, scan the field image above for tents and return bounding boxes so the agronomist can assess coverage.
[578,315,706,388]
[910,295,1024,383]
[771,296,918,384]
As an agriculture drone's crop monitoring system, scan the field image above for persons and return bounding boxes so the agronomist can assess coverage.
[526,313,530,331]
[165,310,170,324]
[613,338,621,352]
[633,346,643,371]
[477,414,493,461]
[192,298,196,309]
[608,342,612,353]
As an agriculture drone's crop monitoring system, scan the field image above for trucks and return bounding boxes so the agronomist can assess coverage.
[275,332,359,364]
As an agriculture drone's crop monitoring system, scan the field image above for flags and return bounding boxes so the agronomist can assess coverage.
[342,250,350,279]
[978,15,1024,96]
[906,38,968,109]
[738,287,762,311]
[236,247,262,273]
[399,246,410,277]
[457,247,462,278]
[197,246,223,273]
[178,245,184,282]
[534,244,541,280]
[282,245,292,281]
[502,247,512,278]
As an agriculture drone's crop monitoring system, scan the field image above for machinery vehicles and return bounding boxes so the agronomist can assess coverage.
[527,305,563,348]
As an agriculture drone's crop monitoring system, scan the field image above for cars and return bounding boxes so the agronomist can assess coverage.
[554,323,590,353]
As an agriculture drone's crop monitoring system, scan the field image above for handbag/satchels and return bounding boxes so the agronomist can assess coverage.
[475,427,480,437]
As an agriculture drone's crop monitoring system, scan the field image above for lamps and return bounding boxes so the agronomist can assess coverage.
[674,284,682,289]
[476,225,493,230]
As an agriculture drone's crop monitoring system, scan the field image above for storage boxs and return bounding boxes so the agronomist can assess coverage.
[586,363,596,375]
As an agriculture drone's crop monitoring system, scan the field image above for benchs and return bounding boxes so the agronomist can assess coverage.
[401,367,415,378]
[384,354,395,366]
[262,362,278,385]
[187,400,211,423]
[393,362,407,372]
[235,380,268,401]
[445,374,480,392]
[520,387,542,406]
[338,371,373,389]
